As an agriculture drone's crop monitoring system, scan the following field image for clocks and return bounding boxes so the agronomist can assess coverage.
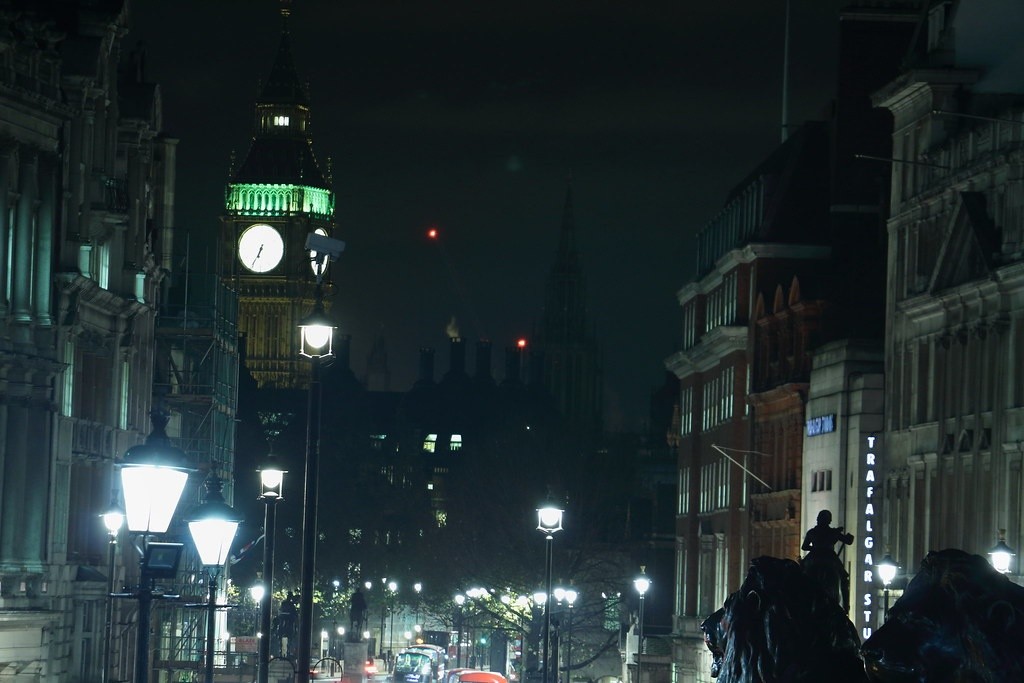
[237,223,285,275]
[309,226,330,279]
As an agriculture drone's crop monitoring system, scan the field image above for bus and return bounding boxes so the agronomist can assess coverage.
[421,630,472,658]
[390,644,508,683]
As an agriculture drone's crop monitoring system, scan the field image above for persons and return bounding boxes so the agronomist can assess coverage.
[802,510,854,614]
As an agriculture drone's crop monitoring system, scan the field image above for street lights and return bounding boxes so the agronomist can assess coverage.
[389,582,398,652]
[182,461,245,682]
[249,580,265,636]
[874,553,902,629]
[294,294,339,683]
[110,407,202,682]
[254,451,290,683]
[414,583,422,624]
[634,573,653,682]
[364,581,372,631]
[535,495,565,683]
[454,594,466,668]
[565,588,577,682]
